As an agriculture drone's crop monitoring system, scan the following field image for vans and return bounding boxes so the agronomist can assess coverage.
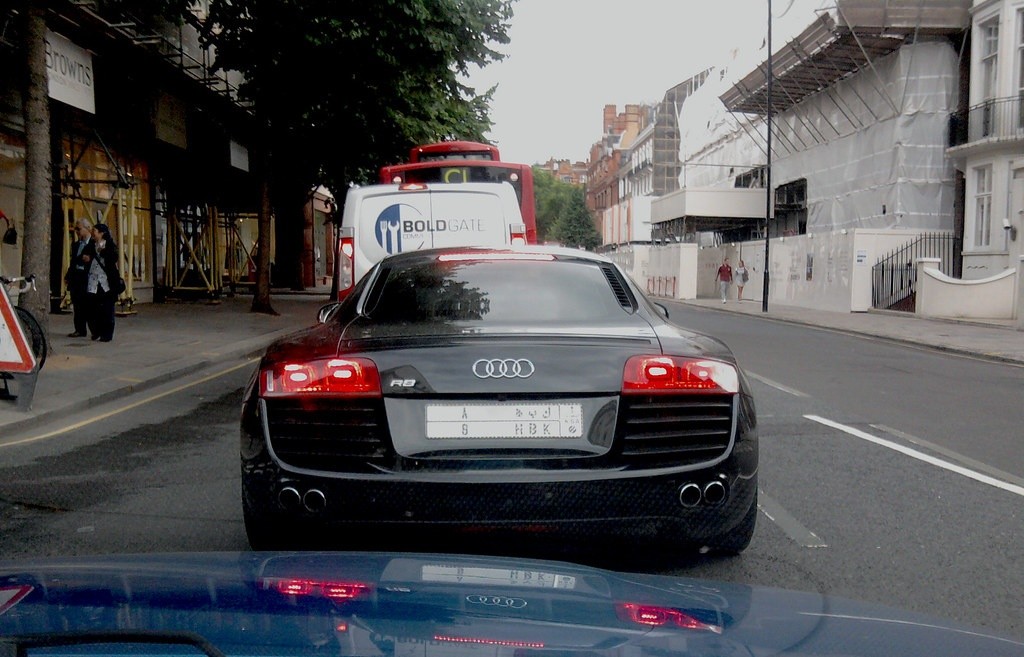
[338,182,528,305]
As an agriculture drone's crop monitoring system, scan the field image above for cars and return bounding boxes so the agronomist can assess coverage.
[240,248,759,560]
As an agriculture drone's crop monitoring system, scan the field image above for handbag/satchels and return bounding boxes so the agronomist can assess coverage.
[114,277,127,295]
[742,267,749,282]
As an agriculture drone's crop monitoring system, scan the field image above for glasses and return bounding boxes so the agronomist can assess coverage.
[74,226,82,232]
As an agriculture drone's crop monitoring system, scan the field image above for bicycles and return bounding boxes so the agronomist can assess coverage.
[0,274,40,359]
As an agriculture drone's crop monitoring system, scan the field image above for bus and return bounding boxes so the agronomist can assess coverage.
[382,141,537,245]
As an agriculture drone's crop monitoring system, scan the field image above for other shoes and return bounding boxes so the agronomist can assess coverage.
[100,336,111,342]
[91,335,99,340]
[68,331,82,338]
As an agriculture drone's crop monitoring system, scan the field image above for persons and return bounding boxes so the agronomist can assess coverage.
[63,218,119,342]
[733,260,749,304]
[715,258,733,305]
